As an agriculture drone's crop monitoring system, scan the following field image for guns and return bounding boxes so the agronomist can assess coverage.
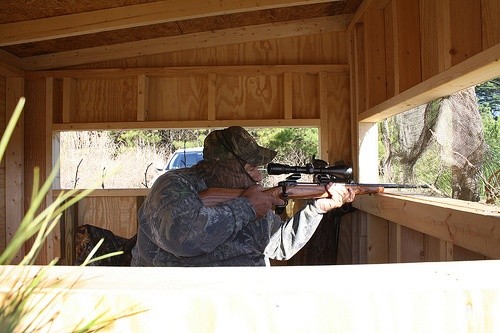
[198,158,439,215]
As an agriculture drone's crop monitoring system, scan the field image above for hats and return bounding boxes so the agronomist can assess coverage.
[203,126,278,166]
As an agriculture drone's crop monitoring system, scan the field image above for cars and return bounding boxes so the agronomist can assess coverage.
[155,146,203,173]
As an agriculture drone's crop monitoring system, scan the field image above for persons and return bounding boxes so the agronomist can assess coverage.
[130,126,355,266]
